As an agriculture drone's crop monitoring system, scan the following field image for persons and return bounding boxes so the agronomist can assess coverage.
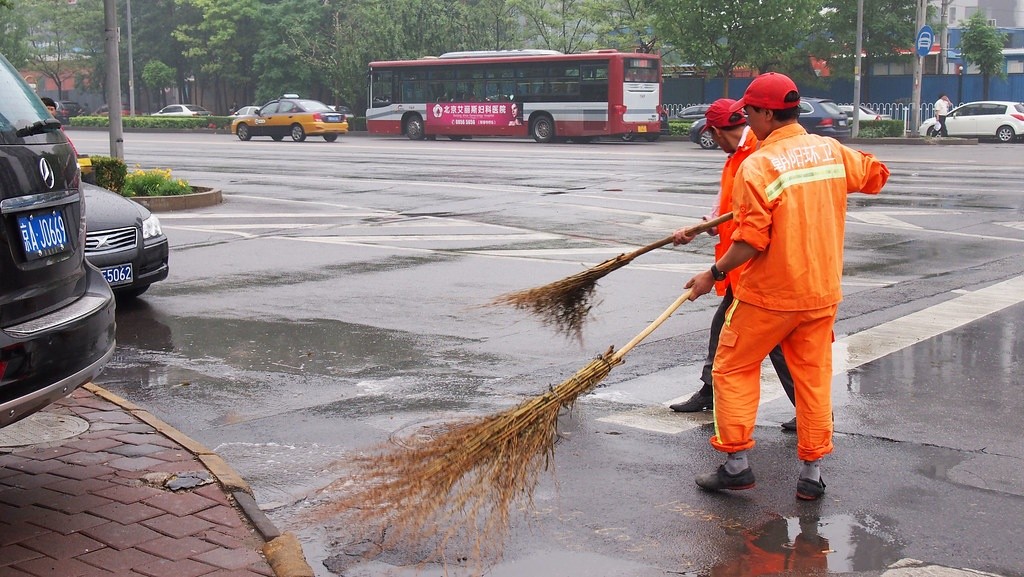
[41,98,56,119]
[693,71,889,500]
[668,99,834,428]
[930,92,953,137]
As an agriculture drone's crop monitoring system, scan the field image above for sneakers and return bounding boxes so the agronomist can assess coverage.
[695,461,755,490]
[796,474,826,499]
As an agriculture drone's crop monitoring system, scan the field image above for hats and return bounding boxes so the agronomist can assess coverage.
[729,72,800,113]
[700,99,747,134]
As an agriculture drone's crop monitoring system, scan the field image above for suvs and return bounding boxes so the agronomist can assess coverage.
[53,101,82,124]
[688,96,852,150]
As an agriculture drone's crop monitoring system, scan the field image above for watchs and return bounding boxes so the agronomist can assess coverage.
[711,265,726,281]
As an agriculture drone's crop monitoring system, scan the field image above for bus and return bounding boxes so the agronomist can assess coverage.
[365,48,663,143]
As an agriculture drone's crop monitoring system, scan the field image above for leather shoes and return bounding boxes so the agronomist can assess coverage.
[781,410,835,431]
[669,391,713,411]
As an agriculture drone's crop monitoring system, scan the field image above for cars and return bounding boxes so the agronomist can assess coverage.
[227,105,262,117]
[669,106,714,120]
[150,104,212,116]
[918,101,1024,143]
[837,104,892,122]
[93,102,141,118]
[0,52,117,428]
[328,105,354,117]
[72,180,171,305]
[231,98,349,143]
